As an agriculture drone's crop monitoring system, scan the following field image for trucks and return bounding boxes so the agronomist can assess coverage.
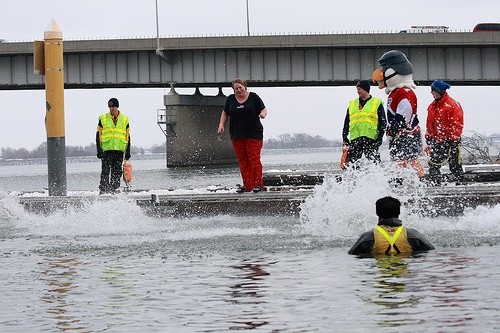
[399,25,450,33]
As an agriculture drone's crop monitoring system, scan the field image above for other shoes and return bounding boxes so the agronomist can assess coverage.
[253,184,261,191]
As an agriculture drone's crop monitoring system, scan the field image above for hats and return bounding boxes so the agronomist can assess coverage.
[108,98,119,107]
[431,79,451,95]
[376,197,400,219]
[356,80,370,93]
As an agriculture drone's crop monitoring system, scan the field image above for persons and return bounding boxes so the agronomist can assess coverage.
[372,50,434,188]
[217,78,267,192]
[348,196,435,255]
[342,80,387,170]
[425,79,469,186]
[96,98,131,194]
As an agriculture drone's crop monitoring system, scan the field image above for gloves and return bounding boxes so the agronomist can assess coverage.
[343,135,350,146]
[97,148,103,159]
[125,153,131,160]
[376,136,383,146]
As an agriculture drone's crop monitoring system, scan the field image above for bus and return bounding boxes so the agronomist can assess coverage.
[472,22,500,32]
